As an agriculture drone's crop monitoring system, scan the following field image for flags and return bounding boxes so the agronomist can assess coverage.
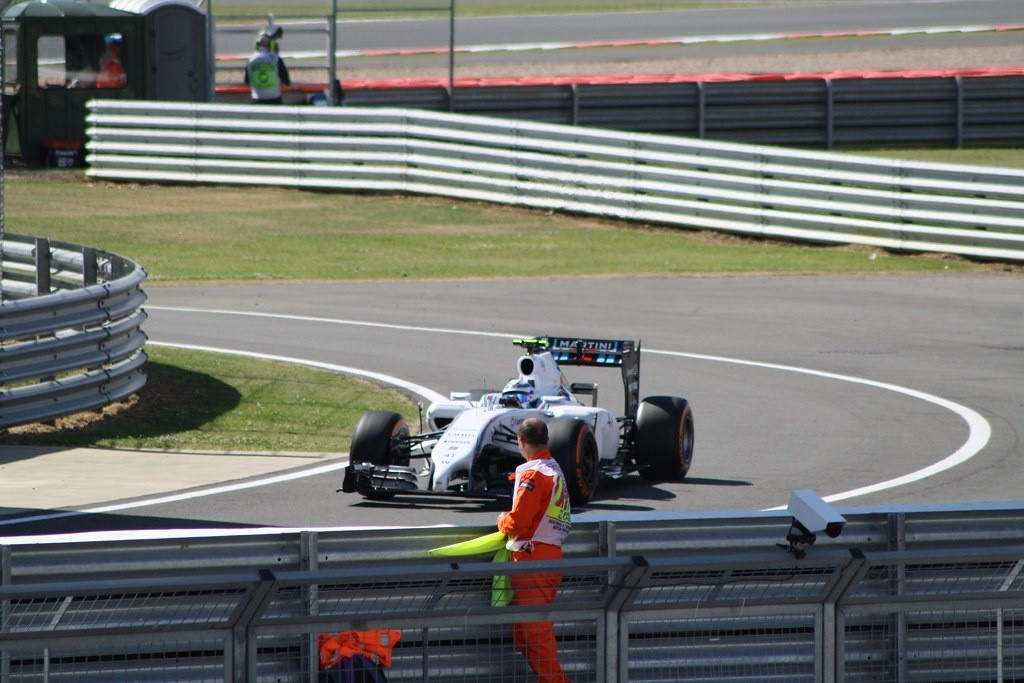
[426,525,517,608]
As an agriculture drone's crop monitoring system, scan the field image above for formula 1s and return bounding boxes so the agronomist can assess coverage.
[337,336,696,508]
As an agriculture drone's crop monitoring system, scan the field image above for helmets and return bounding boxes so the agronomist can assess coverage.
[501,380,535,405]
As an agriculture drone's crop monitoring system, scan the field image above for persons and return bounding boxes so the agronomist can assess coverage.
[497,417,569,683]
[96,41,127,88]
[500,380,539,408]
[243,33,291,104]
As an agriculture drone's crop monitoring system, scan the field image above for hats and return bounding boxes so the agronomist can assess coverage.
[258,36,270,46]
[104,32,123,43]
